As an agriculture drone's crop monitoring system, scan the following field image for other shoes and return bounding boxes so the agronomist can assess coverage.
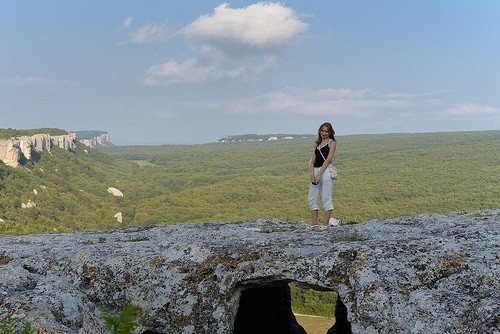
[307,225,319,231]
[319,225,329,230]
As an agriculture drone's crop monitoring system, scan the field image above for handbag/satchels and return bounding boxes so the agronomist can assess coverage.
[329,165,338,180]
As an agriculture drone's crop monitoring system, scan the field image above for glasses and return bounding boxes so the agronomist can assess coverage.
[321,130,330,133]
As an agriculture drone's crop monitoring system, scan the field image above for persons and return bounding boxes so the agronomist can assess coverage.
[307,122,337,228]
